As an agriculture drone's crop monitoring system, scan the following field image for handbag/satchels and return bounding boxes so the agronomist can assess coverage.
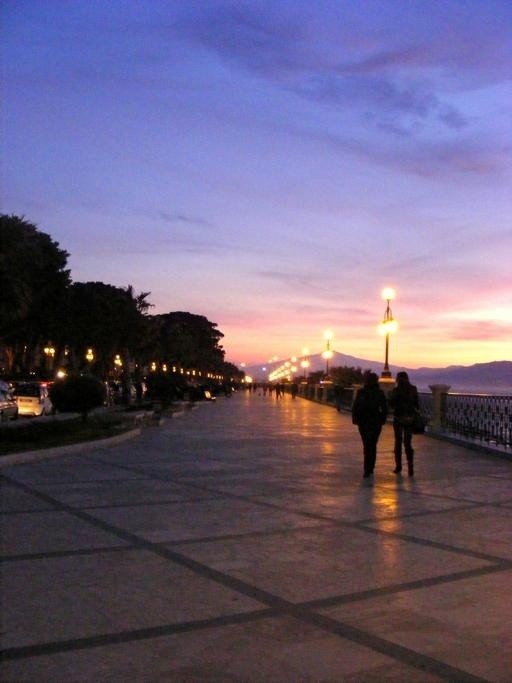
[412,414,427,434]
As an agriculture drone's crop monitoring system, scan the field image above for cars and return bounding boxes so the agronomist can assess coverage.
[0,378,57,423]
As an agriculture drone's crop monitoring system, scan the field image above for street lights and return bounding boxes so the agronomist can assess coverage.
[321,327,334,380]
[376,286,399,378]
[266,348,312,383]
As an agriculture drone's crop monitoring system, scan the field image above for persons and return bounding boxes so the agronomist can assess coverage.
[350,371,389,478]
[333,380,345,411]
[388,372,420,478]
[247,378,299,400]
